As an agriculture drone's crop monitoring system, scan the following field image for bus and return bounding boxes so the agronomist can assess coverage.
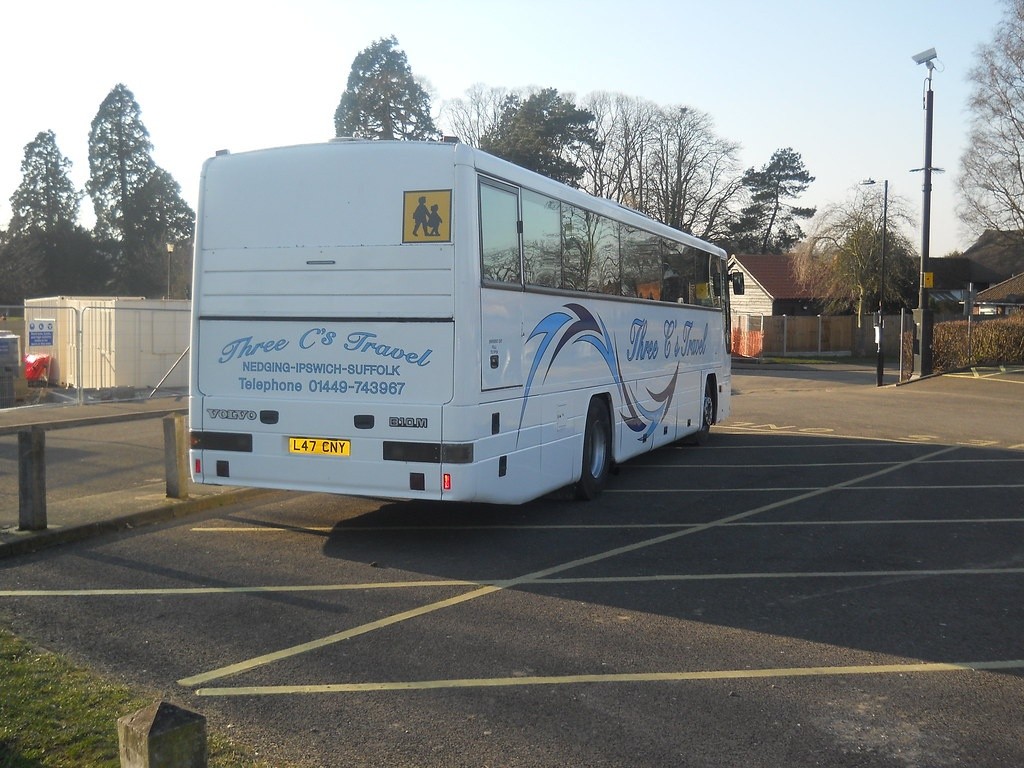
[188,139,745,505]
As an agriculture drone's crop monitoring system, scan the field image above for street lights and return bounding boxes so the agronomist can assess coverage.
[860,178,888,387]
[166,241,176,299]
